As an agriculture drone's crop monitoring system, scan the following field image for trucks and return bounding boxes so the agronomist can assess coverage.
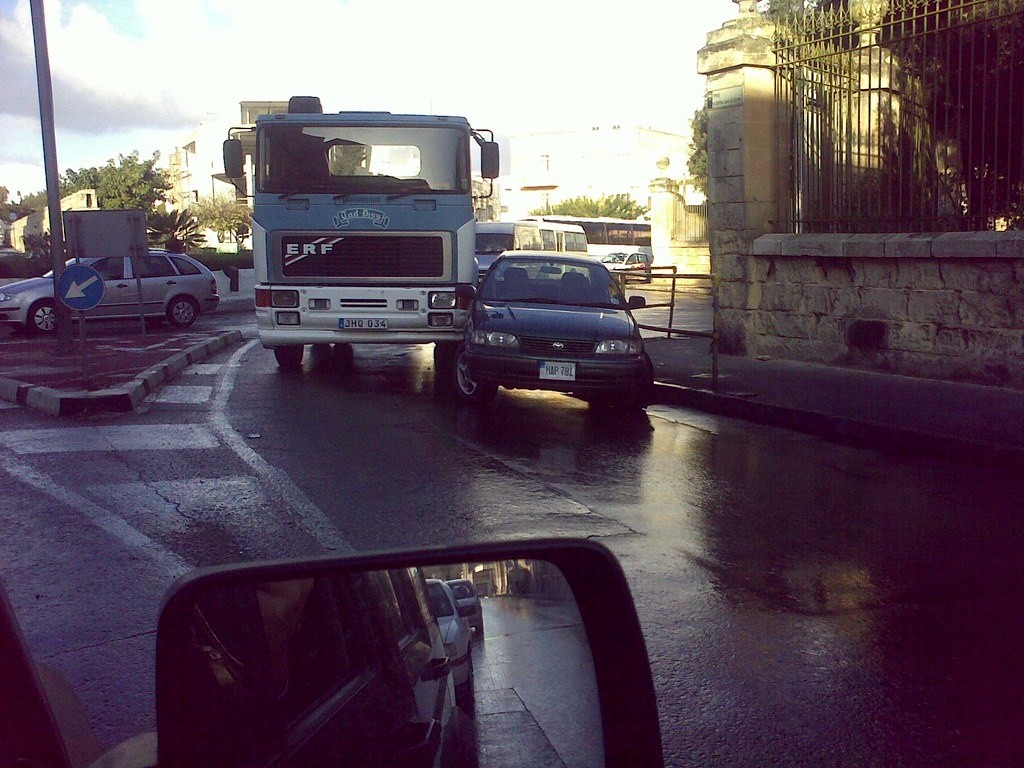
[222,95,501,366]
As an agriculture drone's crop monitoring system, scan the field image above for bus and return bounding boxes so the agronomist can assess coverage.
[525,213,651,262]
[476,221,590,283]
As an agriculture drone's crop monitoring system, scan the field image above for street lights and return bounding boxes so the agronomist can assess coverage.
[539,153,551,212]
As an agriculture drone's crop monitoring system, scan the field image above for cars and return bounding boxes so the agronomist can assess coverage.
[599,251,654,284]
[443,249,655,415]
[423,577,478,689]
[443,580,487,640]
[0,248,221,333]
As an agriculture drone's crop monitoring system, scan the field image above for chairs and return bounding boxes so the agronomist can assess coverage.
[497,268,532,299]
[560,272,595,303]
